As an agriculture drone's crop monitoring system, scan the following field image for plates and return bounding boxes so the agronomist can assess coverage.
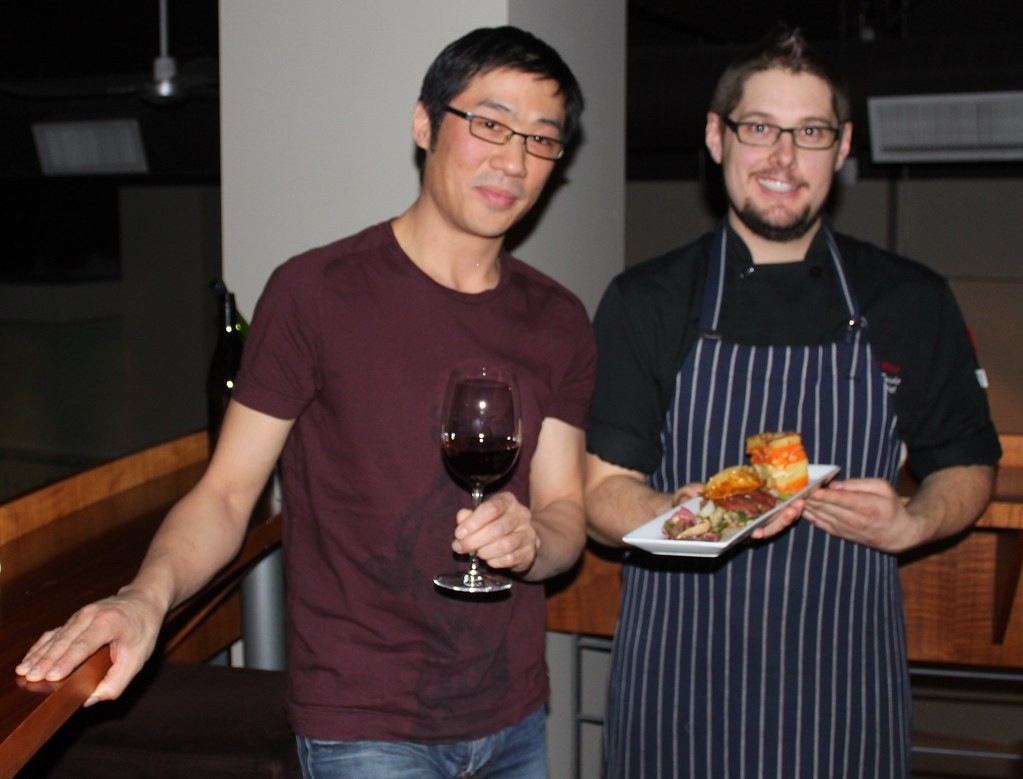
[621,464,842,558]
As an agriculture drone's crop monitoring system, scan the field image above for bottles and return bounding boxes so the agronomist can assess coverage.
[204,277,280,533]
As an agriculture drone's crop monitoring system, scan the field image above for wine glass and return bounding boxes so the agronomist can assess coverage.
[431,361,524,594]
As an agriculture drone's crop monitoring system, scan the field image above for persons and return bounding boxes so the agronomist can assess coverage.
[584,35,1002,779]
[16,25,598,778]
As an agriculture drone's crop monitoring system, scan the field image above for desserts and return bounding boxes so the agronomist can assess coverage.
[745,431,809,500]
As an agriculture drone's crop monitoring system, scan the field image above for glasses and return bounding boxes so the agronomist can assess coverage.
[432,102,568,161]
[722,116,843,150]
